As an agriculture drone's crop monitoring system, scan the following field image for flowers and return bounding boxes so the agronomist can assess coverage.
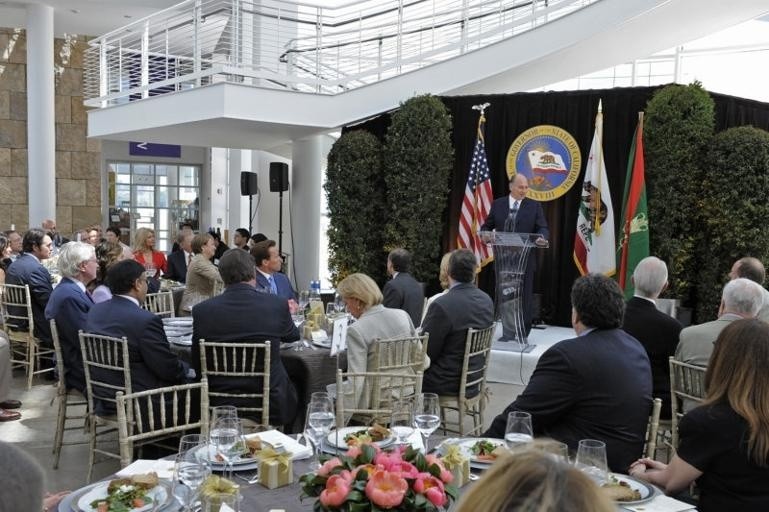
[298,430,457,512]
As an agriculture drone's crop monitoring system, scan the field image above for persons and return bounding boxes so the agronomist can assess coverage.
[480,174,550,344]
[1,223,494,459]
[451,255,767,511]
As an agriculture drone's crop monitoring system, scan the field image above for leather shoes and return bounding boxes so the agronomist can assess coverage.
[0,399,21,421]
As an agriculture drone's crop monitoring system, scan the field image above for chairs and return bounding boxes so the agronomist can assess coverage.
[0,283,496,486]
[642,398,663,460]
[668,355,707,501]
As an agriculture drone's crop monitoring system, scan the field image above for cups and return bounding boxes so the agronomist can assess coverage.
[541,439,570,467]
[503,410,533,454]
[390,402,414,443]
[573,439,609,492]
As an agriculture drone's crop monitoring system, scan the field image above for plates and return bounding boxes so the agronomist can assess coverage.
[316,425,403,456]
[161,316,194,346]
[196,439,272,472]
[608,470,655,504]
[56,477,190,511]
[445,436,508,470]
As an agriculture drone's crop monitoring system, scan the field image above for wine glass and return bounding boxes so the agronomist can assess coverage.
[415,394,441,459]
[172,405,247,512]
[303,390,334,475]
[292,287,346,351]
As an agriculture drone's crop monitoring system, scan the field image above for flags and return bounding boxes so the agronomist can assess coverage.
[616,121,651,304]
[456,116,495,278]
[572,112,617,278]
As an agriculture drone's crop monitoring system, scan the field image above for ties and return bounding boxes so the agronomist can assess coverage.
[514,202,518,213]
[269,277,278,295]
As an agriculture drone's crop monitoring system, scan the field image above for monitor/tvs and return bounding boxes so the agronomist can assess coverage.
[241,172,257,196]
[269,162,288,192]
